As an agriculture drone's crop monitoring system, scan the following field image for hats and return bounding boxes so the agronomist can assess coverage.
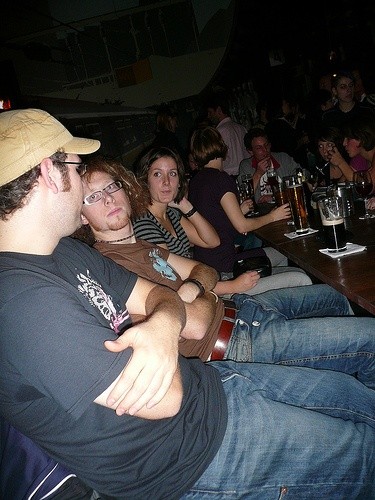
[0,106,103,187]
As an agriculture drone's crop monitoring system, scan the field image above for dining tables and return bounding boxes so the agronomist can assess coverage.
[247,209,375,316]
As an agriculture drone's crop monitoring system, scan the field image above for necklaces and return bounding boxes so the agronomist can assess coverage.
[94,232,136,244]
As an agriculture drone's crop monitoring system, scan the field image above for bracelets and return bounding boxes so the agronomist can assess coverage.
[183,278,205,297]
[209,290,218,303]
[183,206,197,218]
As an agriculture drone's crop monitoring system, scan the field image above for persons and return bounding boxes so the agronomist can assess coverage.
[132,145,312,300]
[0,108,375,500]
[76,160,375,390]
[154,69,375,218]
[0,96,10,112]
[186,126,291,278]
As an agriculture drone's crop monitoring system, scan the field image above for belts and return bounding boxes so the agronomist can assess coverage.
[212,295,237,360]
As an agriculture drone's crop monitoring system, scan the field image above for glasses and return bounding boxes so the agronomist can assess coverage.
[87,178,125,205]
[251,141,271,151]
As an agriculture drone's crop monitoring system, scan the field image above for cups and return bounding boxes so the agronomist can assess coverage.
[267,169,280,188]
[284,177,309,235]
[241,174,254,201]
[318,196,347,253]
[272,182,287,207]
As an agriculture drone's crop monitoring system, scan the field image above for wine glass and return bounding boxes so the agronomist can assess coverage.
[315,148,343,175]
[353,170,375,220]
[239,183,259,217]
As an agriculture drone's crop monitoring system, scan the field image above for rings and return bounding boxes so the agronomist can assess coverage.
[248,203,251,206]
